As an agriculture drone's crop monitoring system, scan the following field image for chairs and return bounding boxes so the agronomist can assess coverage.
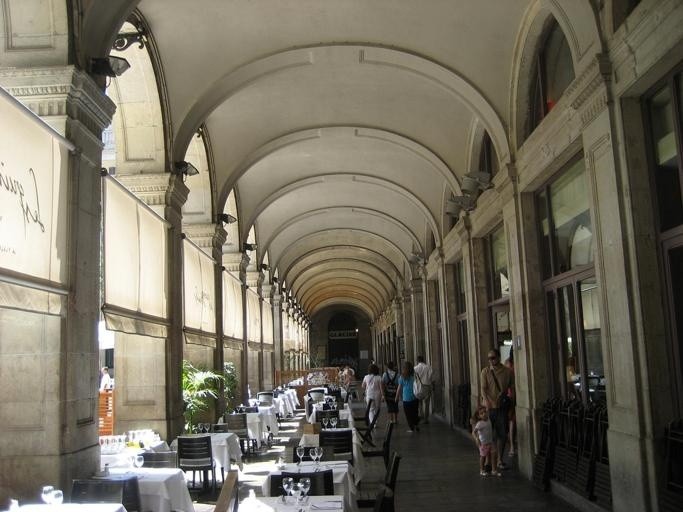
[530,394,612,512]
[1,368,399,512]
[659,416,683,512]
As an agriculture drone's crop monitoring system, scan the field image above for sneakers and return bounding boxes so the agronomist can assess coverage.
[406,428,420,433]
[480,461,510,477]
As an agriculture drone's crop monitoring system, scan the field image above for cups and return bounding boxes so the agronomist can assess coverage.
[41,484,63,506]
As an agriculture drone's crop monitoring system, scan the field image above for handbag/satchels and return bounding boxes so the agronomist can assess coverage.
[413,372,428,401]
[496,391,514,412]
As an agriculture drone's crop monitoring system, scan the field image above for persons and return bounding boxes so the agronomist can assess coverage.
[362,364,384,428]
[395,362,420,432]
[339,364,354,385]
[382,361,399,424]
[480,348,516,470]
[100,366,110,409]
[413,356,436,425]
[504,357,516,457]
[471,406,502,478]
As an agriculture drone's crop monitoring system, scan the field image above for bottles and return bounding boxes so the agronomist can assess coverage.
[104,464,110,476]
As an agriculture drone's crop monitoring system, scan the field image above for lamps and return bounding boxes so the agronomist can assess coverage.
[217,212,236,225]
[459,173,494,196]
[91,54,131,79]
[446,197,475,217]
[408,251,427,266]
[175,161,199,177]
[246,242,313,326]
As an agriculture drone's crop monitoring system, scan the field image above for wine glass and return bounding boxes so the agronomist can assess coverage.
[295,447,323,470]
[135,456,143,473]
[236,406,246,413]
[196,423,211,434]
[283,477,311,509]
[99,435,126,455]
[323,401,340,430]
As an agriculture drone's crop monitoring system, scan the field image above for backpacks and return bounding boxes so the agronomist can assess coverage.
[384,371,398,403]
